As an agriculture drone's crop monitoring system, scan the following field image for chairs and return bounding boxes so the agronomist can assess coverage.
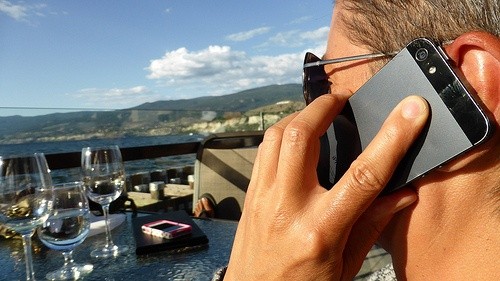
[192,131,266,220]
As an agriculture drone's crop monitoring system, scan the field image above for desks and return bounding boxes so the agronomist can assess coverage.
[123,183,194,212]
[0,207,239,281]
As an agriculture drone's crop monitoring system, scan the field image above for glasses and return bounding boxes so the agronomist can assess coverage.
[302,36,457,106]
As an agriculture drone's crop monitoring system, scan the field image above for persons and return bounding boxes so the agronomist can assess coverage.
[194,0,500,281]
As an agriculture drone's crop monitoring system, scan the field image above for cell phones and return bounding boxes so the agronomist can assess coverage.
[316,36,492,198]
[141,219,192,239]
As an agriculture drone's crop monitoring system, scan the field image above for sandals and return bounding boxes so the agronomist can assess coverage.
[193,193,217,220]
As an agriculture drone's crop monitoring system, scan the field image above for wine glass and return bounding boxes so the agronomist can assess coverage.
[33,181,94,281]
[0,152,55,280]
[80,145,129,260]
